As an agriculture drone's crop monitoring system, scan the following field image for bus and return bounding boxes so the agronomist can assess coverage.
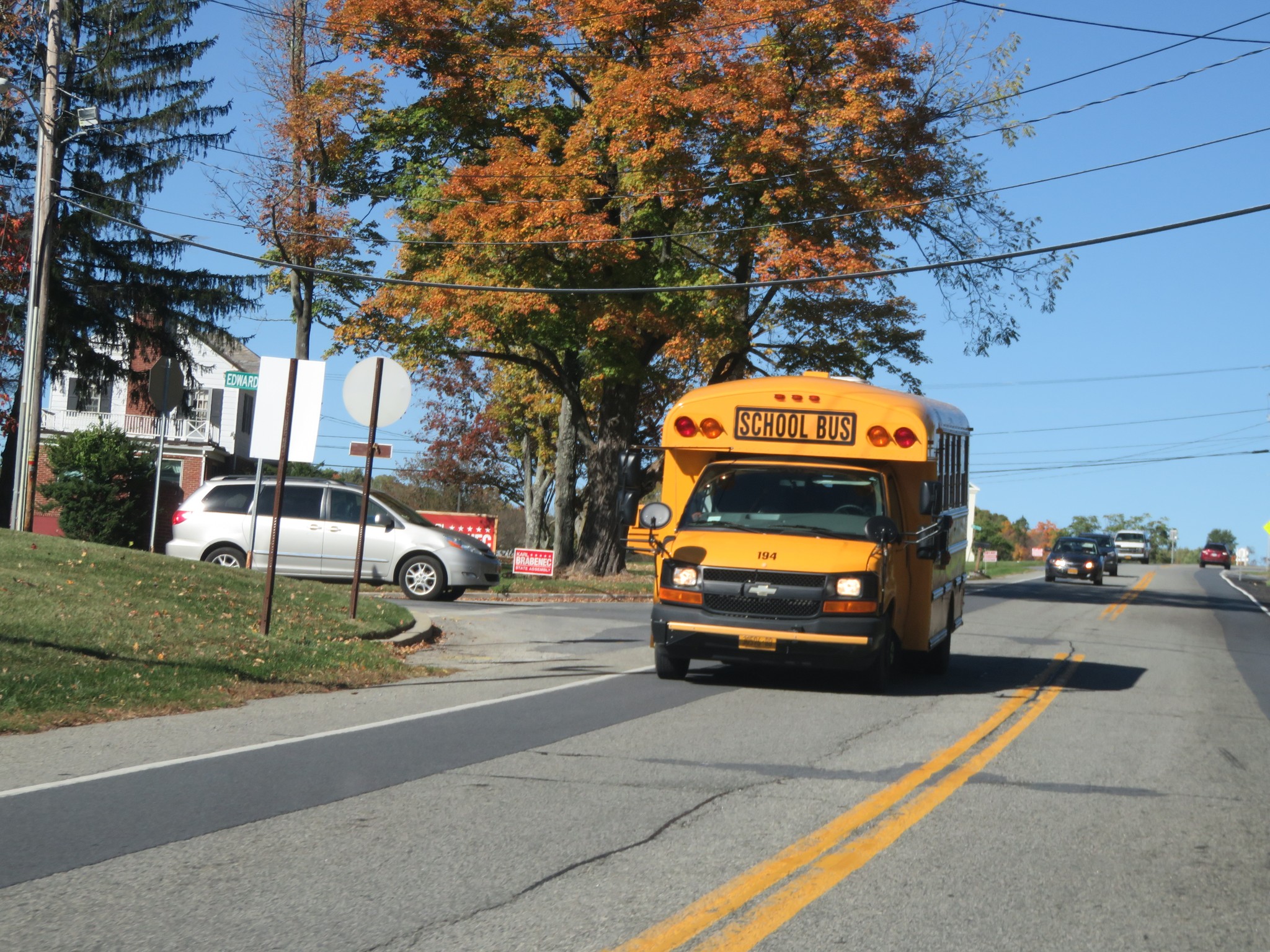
[611,369,974,692]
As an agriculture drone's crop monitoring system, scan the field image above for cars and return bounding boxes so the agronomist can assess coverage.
[1199,542,1231,569]
[1044,537,1107,585]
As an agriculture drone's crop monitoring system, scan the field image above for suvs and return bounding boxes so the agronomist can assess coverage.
[1112,530,1151,564]
[1078,533,1121,576]
[166,475,500,600]
